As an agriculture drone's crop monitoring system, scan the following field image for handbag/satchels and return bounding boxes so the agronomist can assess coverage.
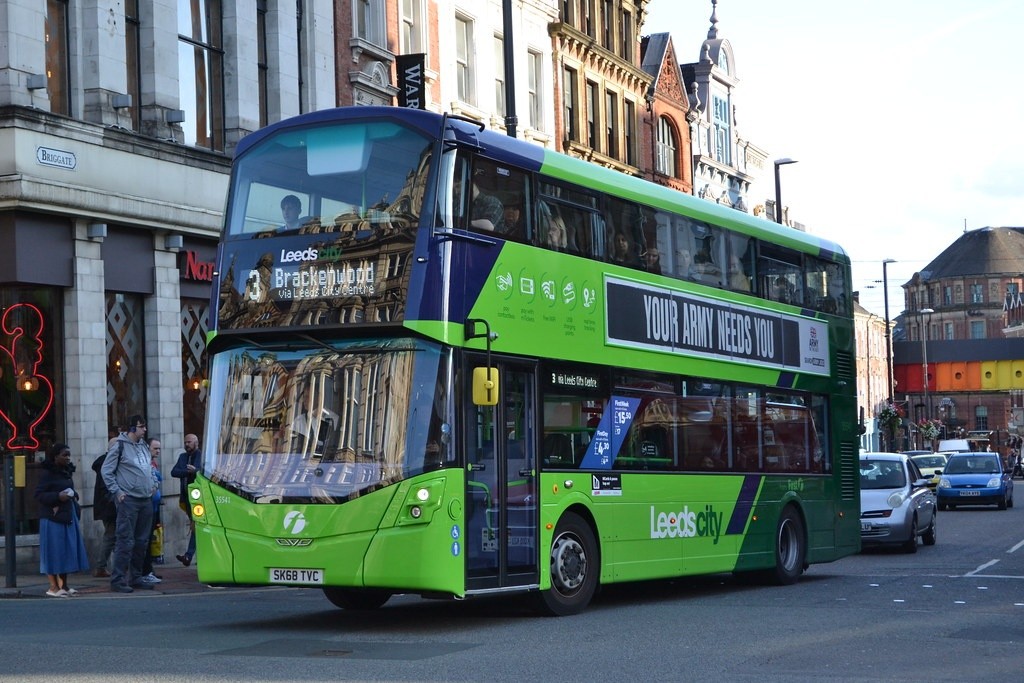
[149,527,165,557]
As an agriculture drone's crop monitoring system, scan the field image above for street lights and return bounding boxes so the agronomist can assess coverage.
[774,157,797,225]
[882,258,899,404]
[919,308,934,420]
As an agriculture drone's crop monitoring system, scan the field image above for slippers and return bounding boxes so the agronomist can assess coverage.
[67,588,80,596]
[46,590,69,598]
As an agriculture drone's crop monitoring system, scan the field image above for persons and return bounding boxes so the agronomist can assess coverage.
[452,171,527,241]
[694,245,714,265]
[647,246,661,275]
[34,443,90,598]
[276,195,309,233]
[547,216,578,254]
[730,255,751,292]
[608,231,634,264]
[273,382,340,452]
[675,248,697,281]
[776,277,847,317]
[91,414,163,593]
[171,433,205,567]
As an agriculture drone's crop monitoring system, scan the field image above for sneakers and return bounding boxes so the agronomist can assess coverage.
[142,572,162,582]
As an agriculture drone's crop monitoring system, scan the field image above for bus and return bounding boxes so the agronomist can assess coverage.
[188,106,864,617]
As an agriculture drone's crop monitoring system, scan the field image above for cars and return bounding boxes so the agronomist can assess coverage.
[935,451,1014,510]
[910,455,947,496]
[899,450,959,463]
[859,451,937,553]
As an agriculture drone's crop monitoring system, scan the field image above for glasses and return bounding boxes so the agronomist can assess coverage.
[135,424,146,429]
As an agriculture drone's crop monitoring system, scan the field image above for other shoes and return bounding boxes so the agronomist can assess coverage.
[176,555,191,567]
[111,582,134,593]
[94,568,110,577]
[129,581,155,590]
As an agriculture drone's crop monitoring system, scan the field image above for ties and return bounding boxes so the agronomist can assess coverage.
[188,456,193,464]
